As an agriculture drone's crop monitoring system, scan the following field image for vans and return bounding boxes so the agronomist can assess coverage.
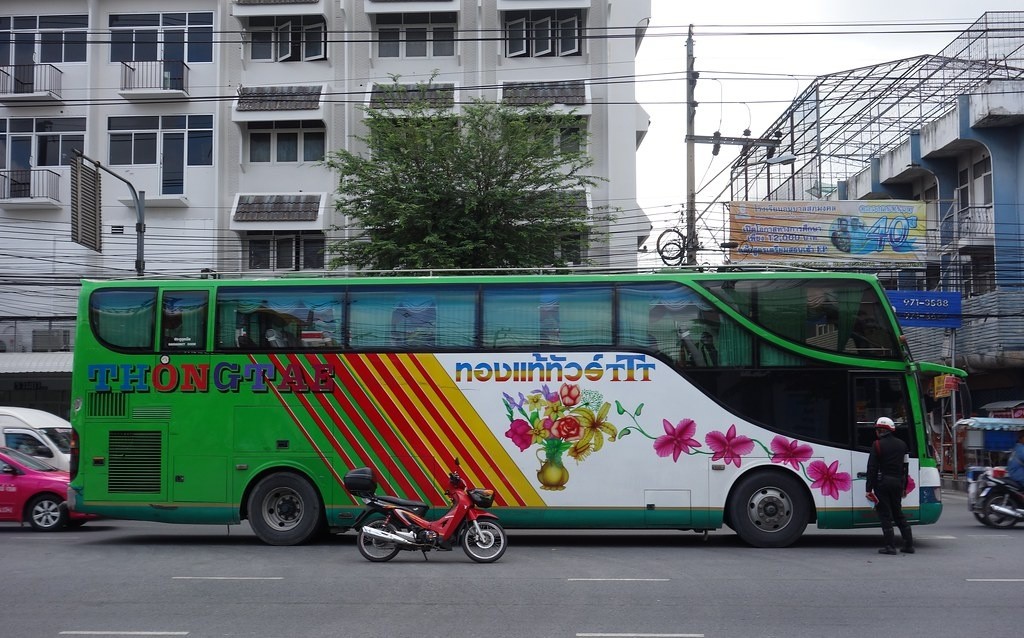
[0,406,73,472]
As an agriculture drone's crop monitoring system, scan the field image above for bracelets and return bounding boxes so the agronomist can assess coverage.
[681,330,690,339]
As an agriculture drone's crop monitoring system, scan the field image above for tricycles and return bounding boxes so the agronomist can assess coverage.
[952,417,1024,524]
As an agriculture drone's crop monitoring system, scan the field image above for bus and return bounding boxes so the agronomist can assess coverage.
[66,271,968,549]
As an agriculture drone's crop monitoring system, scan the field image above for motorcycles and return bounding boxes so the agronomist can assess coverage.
[343,456,508,564]
[975,470,1024,529]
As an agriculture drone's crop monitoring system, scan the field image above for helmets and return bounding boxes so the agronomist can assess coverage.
[873,416,896,431]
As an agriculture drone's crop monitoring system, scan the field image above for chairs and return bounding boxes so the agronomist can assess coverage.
[156,324,707,369]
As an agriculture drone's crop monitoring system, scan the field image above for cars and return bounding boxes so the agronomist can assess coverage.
[0,446,100,531]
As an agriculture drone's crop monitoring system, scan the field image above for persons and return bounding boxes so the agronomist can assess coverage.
[1007,430,1024,491]
[649,322,708,366]
[831,218,851,252]
[19,437,38,456]
[849,218,867,253]
[865,417,914,555]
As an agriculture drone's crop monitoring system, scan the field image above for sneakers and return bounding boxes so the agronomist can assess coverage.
[879,546,896,555]
[900,548,914,553]
